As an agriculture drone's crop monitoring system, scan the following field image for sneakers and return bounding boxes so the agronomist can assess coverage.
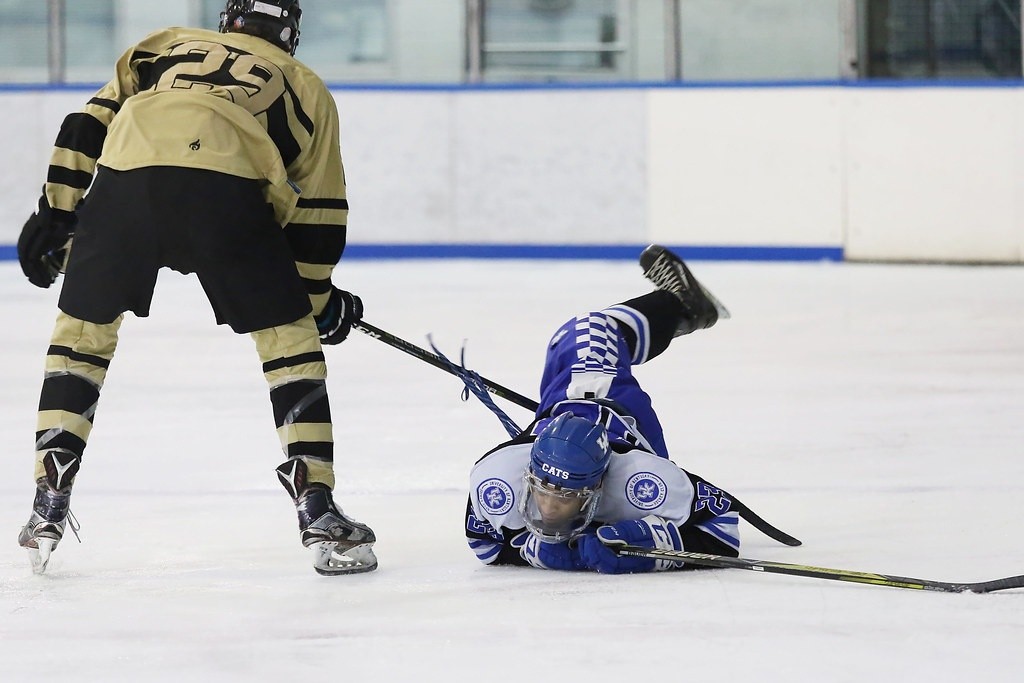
[640,243,732,329]
[276,458,379,576]
[17,452,82,572]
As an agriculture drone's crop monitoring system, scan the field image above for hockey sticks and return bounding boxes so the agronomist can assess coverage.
[350,319,804,548]
[567,533,1024,597]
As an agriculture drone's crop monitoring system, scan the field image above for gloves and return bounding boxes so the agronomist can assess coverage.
[17,183,78,289]
[524,533,579,572]
[577,516,686,575]
[314,287,363,346]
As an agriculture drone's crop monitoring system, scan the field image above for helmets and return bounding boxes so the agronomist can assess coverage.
[530,412,611,491]
[218,0,302,56]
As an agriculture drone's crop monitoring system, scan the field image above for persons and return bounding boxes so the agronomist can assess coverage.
[463,244,742,575]
[17,0,381,576]
